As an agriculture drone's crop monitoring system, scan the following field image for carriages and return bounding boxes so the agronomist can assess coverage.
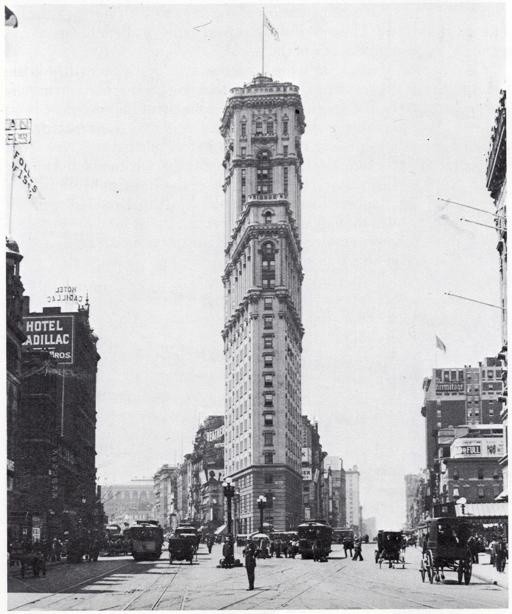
[416,515,475,585]
[374,528,406,570]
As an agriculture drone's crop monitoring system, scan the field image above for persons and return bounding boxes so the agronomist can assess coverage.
[468,531,508,573]
[206,531,215,552]
[261,528,302,563]
[343,537,364,561]
[242,540,259,591]
[8,524,129,582]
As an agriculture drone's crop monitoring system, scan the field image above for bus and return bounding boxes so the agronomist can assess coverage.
[296,518,332,558]
[173,522,199,551]
[131,521,163,560]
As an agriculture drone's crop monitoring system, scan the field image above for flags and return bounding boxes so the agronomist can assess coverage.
[5,6,19,28]
[436,334,448,353]
[265,9,281,42]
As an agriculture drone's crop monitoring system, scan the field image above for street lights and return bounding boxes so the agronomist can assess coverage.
[256,493,266,532]
[220,474,242,568]
